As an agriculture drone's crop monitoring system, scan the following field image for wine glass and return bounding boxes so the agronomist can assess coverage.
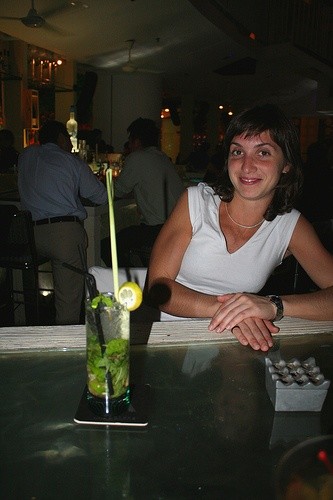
[78,139,87,160]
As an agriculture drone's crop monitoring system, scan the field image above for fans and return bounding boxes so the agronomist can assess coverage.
[0,0,77,40]
[88,40,176,74]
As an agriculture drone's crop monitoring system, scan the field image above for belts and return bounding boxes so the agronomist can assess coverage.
[32,216,80,226]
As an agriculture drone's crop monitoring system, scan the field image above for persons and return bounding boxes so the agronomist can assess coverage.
[115,117,185,247]
[296,143,333,223]
[146,103,333,352]
[18,122,106,325]
[0,129,16,170]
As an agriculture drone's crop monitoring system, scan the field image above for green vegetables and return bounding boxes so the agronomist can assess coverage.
[86,293,129,395]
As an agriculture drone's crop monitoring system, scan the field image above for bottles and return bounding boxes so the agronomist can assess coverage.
[66,105,78,137]
[87,153,124,179]
[31,100,39,128]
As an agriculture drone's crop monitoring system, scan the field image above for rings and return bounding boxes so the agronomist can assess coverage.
[231,325,240,333]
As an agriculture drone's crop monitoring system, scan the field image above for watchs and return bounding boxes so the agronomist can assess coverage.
[266,295,283,321]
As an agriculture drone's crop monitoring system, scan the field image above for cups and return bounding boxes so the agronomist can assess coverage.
[273,434,333,500]
[84,292,131,408]
[112,169,119,179]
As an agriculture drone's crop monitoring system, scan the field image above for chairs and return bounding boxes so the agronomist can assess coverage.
[0,205,55,326]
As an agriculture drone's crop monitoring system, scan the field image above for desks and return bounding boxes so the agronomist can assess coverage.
[0,190,141,326]
[0,331,333,500]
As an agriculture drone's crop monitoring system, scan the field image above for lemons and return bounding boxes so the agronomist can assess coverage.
[119,282,142,309]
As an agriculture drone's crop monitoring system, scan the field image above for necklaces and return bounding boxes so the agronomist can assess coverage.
[225,202,265,229]
